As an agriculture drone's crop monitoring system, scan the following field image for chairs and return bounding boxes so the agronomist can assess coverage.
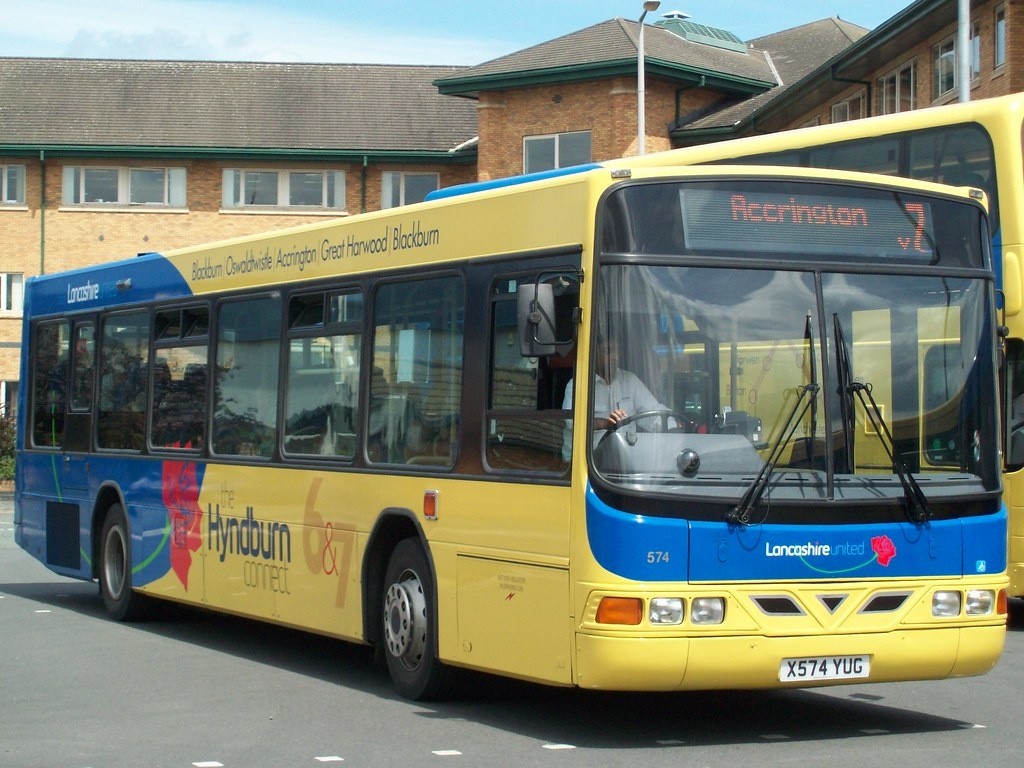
[183,363,219,382]
[944,171,986,185]
[405,426,452,465]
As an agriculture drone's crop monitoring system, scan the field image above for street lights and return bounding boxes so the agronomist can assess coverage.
[634,1,663,156]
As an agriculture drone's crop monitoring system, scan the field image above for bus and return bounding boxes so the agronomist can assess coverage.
[10,163,1011,706]
[430,88,1021,628]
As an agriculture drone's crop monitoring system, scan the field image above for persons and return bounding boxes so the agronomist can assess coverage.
[39,340,210,449]
[561,331,680,463]
